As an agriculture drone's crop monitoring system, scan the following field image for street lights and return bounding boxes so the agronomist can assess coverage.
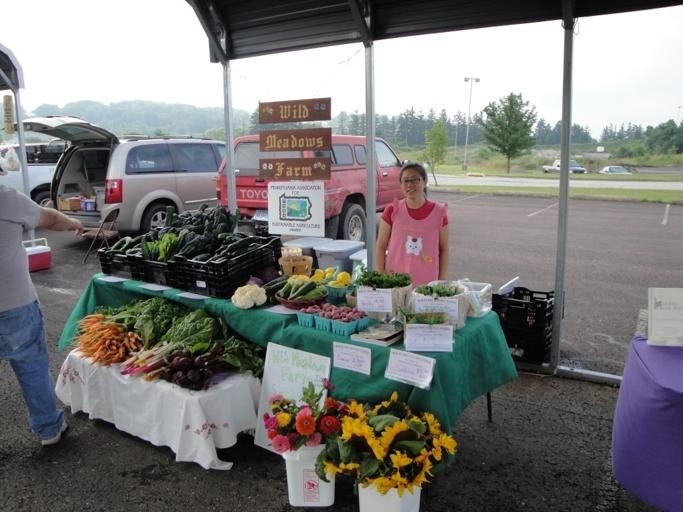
[461,76,480,169]
[675,103,682,129]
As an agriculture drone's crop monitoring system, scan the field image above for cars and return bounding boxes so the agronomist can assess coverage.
[599,165,631,175]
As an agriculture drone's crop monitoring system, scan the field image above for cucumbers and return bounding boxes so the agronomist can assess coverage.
[263,276,326,302]
[110,203,259,265]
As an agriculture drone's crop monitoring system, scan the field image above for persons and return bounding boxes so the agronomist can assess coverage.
[0,182,86,450]
[372,162,449,283]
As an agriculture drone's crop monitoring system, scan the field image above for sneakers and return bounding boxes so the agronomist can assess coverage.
[39,419,70,447]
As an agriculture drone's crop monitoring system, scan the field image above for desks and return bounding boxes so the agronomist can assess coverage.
[54,345,262,472]
[89,273,499,497]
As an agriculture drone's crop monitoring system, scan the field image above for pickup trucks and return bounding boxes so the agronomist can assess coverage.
[542,159,588,175]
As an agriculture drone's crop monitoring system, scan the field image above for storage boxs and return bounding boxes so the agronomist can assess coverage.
[22,237,52,273]
[428,280,493,318]
[85,200,96,212]
[61,197,81,210]
[348,248,388,281]
[282,236,334,269]
[313,239,366,279]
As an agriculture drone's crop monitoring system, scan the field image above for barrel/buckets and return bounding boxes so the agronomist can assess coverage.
[278,256,313,278]
[84,198,96,211]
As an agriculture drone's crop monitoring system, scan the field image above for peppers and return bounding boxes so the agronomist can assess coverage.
[309,267,352,287]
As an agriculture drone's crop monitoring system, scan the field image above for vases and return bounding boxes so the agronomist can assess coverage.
[357,477,422,512]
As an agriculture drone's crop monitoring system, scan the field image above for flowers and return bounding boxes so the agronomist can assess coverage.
[315,390,459,498]
[263,377,359,454]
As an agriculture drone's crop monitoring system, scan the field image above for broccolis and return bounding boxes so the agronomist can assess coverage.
[231,285,267,310]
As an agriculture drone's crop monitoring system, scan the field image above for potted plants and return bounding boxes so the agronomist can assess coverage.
[355,266,412,322]
[404,312,450,349]
[409,280,469,330]
[295,266,369,337]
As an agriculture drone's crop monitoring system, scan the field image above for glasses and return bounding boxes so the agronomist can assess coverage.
[399,177,424,184]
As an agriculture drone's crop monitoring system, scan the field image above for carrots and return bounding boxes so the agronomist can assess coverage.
[74,312,143,370]
